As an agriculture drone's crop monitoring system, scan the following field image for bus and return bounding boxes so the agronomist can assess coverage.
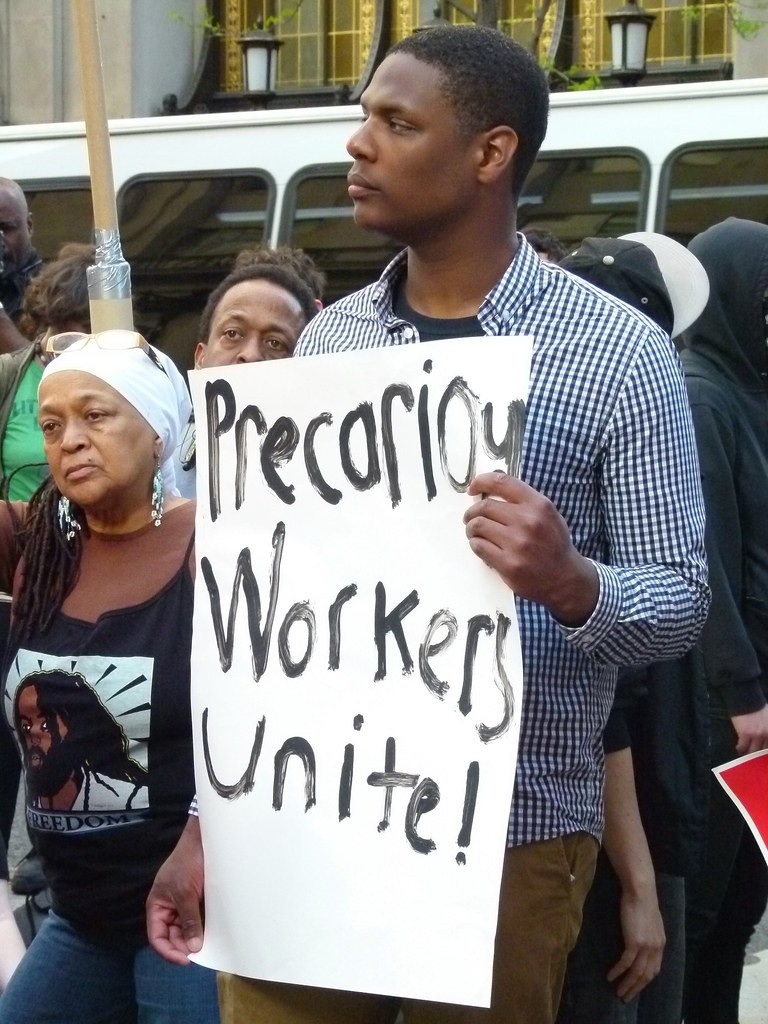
[1,77,767,375]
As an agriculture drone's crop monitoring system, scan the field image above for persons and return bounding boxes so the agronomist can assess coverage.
[0,331,219,1024]
[144,24,710,1024]
[0,176,325,1024]
[523,215,767,1024]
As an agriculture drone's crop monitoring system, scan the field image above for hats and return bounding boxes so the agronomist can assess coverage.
[559,231,710,340]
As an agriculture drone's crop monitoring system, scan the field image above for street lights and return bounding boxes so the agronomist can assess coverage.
[235,22,284,111]
[604,0,657,88]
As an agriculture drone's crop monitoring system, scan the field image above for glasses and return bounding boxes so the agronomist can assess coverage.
[46,330,167,372]
